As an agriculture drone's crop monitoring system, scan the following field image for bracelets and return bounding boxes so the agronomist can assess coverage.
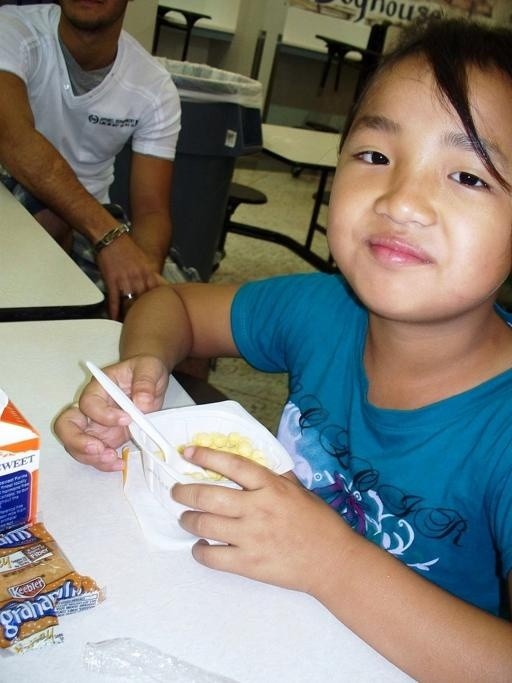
[91,224,128,262]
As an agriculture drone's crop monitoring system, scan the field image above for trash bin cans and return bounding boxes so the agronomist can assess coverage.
[107,54,263,284]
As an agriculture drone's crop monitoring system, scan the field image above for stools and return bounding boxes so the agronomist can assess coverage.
[212,183,268,276]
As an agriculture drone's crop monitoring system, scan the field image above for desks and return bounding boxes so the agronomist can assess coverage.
[0,319,416,683]
[2,181,105,318]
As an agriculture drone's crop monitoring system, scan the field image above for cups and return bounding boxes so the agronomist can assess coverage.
[122,394,298,523]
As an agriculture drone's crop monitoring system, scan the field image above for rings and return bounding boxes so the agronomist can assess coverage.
[69,401,95,423]
[120,292,135,300]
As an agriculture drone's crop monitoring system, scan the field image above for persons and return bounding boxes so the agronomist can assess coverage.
[0,0,212,387]
[53,7,512,683]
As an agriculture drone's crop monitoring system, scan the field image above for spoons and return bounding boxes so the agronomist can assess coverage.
[84,357,211,481]
